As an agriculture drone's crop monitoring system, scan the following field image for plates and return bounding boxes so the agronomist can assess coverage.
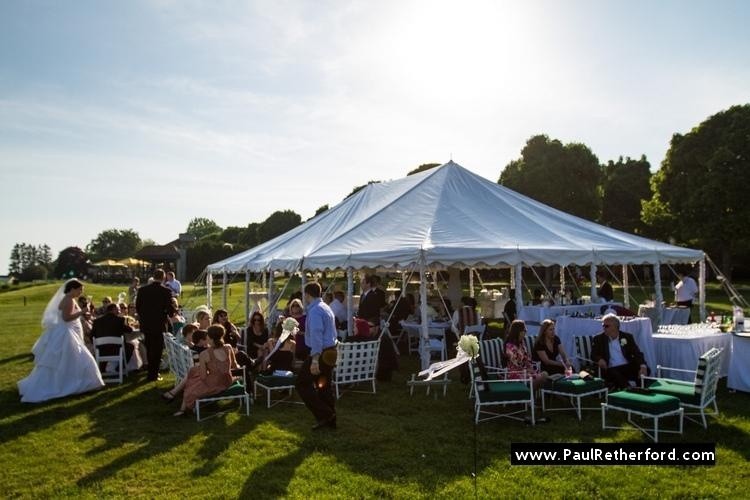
[480,287,507,302]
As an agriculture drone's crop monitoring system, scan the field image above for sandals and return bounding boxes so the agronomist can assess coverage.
[161,389,179,399]
[173,408,190,417]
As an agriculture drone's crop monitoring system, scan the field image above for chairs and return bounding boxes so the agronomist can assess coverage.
[83,281,750,444]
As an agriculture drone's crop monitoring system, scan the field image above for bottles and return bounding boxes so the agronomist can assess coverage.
[707,306,745,332]
[538,290,591,307]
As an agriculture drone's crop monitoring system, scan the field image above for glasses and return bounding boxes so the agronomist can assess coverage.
[253,318,261,322]
[521,328,528,332]
[121,306,127,310]
[103,300,111,304]
[601,323,612,329]
[291,304,299,307]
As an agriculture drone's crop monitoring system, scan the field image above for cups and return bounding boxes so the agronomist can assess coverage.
[564,365,572,378]
[86,295,93,304]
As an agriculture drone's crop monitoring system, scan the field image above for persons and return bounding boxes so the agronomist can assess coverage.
[15,269,699,431]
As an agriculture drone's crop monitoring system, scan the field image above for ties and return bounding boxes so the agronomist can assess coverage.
[359,290,365,304]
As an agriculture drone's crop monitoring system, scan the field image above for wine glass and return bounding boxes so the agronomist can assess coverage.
[656,322,721,338]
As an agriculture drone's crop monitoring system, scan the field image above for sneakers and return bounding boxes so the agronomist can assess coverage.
[311,413,336,430]
